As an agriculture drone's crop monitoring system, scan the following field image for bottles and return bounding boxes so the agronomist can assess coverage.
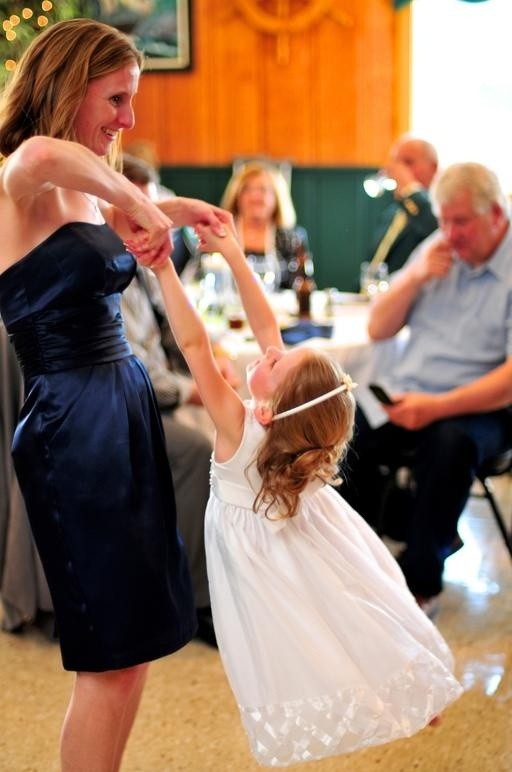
[290,236,315,319]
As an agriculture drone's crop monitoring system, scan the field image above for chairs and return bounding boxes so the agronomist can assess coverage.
[476,447,510,555]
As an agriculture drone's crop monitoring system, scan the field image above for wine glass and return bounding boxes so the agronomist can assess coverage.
[361,262,387,306]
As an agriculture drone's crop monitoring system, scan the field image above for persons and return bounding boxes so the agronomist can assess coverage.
[124,218,469,769]
[0,16,235,771]
[1,139,510,650]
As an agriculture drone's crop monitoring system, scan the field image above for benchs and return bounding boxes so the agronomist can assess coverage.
[159,166,434,293]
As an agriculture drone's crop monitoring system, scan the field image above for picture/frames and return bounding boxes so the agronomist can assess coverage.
[99,1,191,72]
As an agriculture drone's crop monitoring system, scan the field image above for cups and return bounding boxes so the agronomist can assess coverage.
[364,172,397,197]
